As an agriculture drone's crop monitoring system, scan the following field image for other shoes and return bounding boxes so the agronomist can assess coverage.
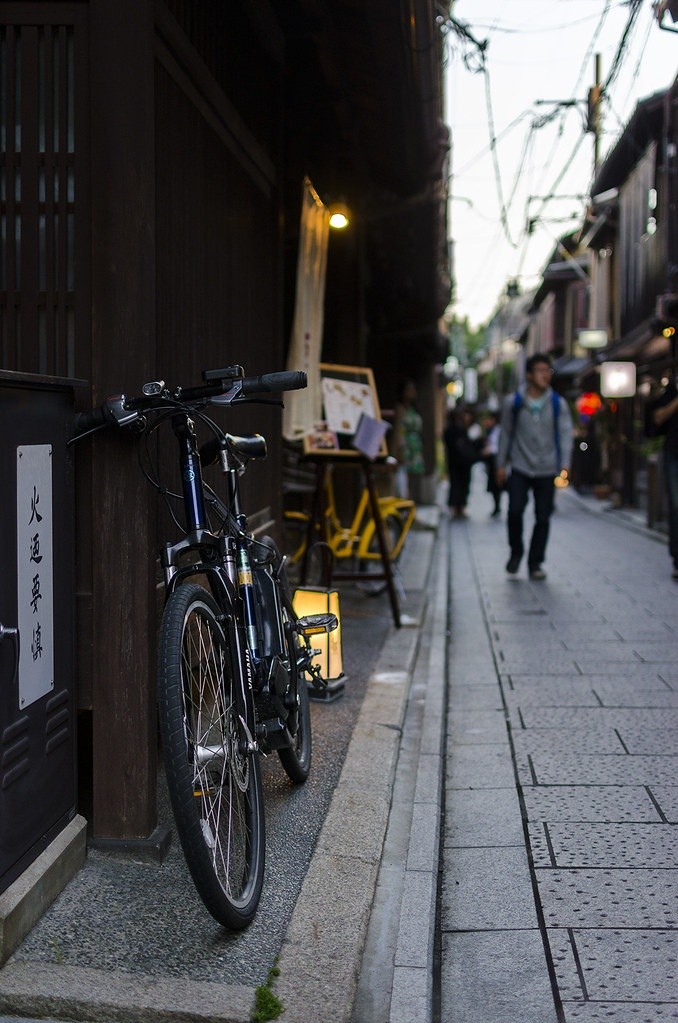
[507,550,523,573]
[529,565,545,579]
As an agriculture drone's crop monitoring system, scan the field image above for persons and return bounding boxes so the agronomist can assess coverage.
[493,352,574,581]
[442,409,485,522]
[389,398,428,500]
[481,413,504,516]
[643,373,678,579]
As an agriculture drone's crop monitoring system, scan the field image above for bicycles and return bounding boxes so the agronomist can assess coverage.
[49,362,339,934]
[273,430,417,598]
